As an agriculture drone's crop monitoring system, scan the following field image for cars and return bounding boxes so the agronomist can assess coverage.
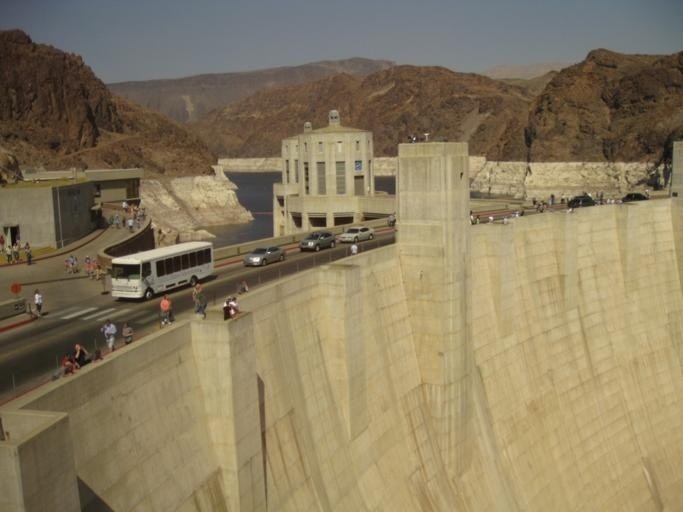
[339,227,374,243]
[299,231,336,251]
[244,245,286,266]
[568,196,595,207]
[622,193,649,202]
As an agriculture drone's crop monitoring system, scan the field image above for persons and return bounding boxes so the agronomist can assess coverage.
[350,241,358,255]
[61,344,90,374]
[109,202,145,231]
[34,289,42,312]
[192,284,239,320]
[122,323,134,344]
[100,319,117,351]
[64,254,101,280]
[469,189,650,225]
[160,294,174,325]
[6,238,31,265]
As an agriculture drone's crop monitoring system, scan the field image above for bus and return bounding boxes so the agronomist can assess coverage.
[111,242,214,301]
